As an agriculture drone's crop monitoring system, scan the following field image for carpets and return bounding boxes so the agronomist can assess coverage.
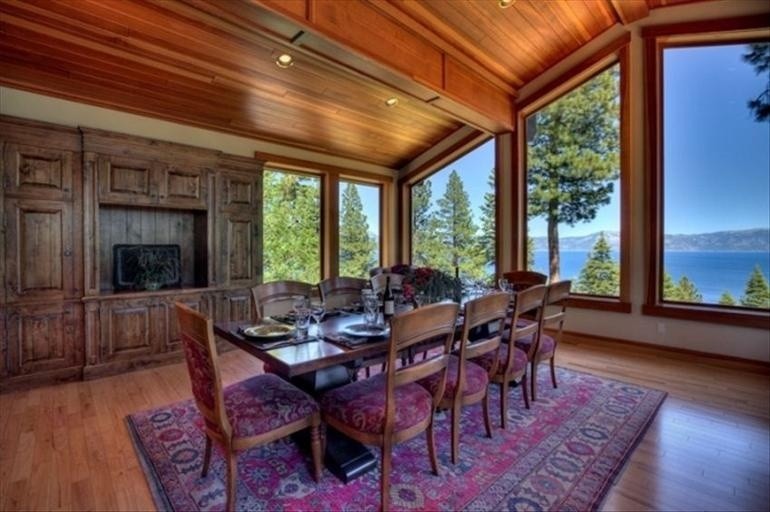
[122,360,670,511]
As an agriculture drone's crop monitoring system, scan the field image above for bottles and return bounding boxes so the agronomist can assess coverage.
[383,274,394,319]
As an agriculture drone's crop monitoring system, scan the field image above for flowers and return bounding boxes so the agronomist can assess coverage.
[399,265,469,307]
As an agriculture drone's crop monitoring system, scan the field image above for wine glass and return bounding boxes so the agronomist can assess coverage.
[460,273,514,303]
[312,301,326,335]
[361,288,379,322]
[293,295,311,337]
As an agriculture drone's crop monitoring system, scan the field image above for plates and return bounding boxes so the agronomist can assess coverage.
[343,323,385,336]
[243,324,297,339]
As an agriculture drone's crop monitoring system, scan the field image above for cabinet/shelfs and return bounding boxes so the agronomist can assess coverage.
[101,291,206,376]
[212,150,265,355]
[1,115,84,394]
[82,128,210,209]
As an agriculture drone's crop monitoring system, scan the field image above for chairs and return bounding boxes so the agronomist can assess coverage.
[415,292,511,465]
[175,302,323,512]
[501,272,549,321]
[321,301,457,512]
[369,266,415,374]
[251,280,314,318]
[317,277,386,377]
[500,278,572,403]
[475,285,546,429]
